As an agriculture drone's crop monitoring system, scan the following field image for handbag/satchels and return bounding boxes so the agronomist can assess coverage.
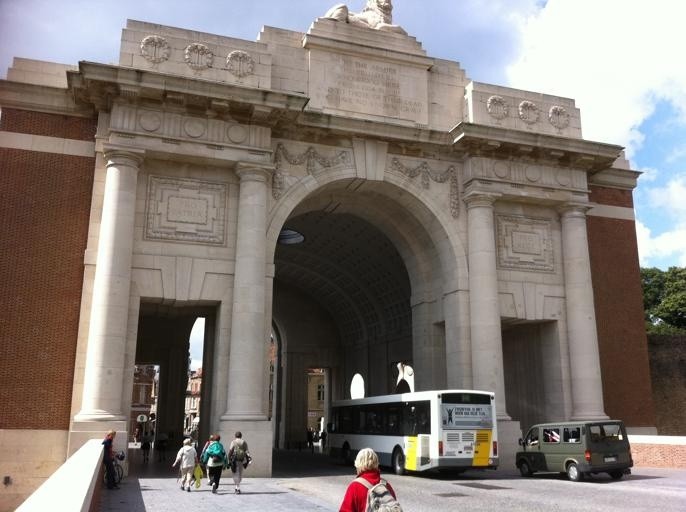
[212,456,220,463]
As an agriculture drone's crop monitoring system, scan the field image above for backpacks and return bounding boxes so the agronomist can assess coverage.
[353,477,403,512]
[233,439,245,461]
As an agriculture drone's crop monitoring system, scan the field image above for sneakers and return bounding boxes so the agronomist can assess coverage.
[180,486,184,490]
[212,482,217,493]
[114,483,120,488]
[233,489,240,494]
[187,487,190,491]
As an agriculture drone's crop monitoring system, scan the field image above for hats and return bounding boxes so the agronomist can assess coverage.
[354,448,378,470]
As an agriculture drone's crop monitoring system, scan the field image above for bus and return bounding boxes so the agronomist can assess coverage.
[326,390,500,475]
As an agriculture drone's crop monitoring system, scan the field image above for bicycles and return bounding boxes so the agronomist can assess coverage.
[104,452,123,486]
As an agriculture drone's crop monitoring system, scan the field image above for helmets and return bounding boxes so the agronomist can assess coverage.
[117,451,125,460]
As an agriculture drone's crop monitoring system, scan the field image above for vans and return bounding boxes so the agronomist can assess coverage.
[516,420,633,481]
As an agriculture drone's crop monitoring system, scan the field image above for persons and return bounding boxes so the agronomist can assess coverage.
[102,430,121,490]
[307,429,313,448]
[172,437,199,492]
[228,432,250,494]
[140,432,152,464]
[132,428,140,446]
[338,447,396,512]
[530,429,550,446]
[321,429,327,450]
[198,433,214,485]
[202,434,230,493]
